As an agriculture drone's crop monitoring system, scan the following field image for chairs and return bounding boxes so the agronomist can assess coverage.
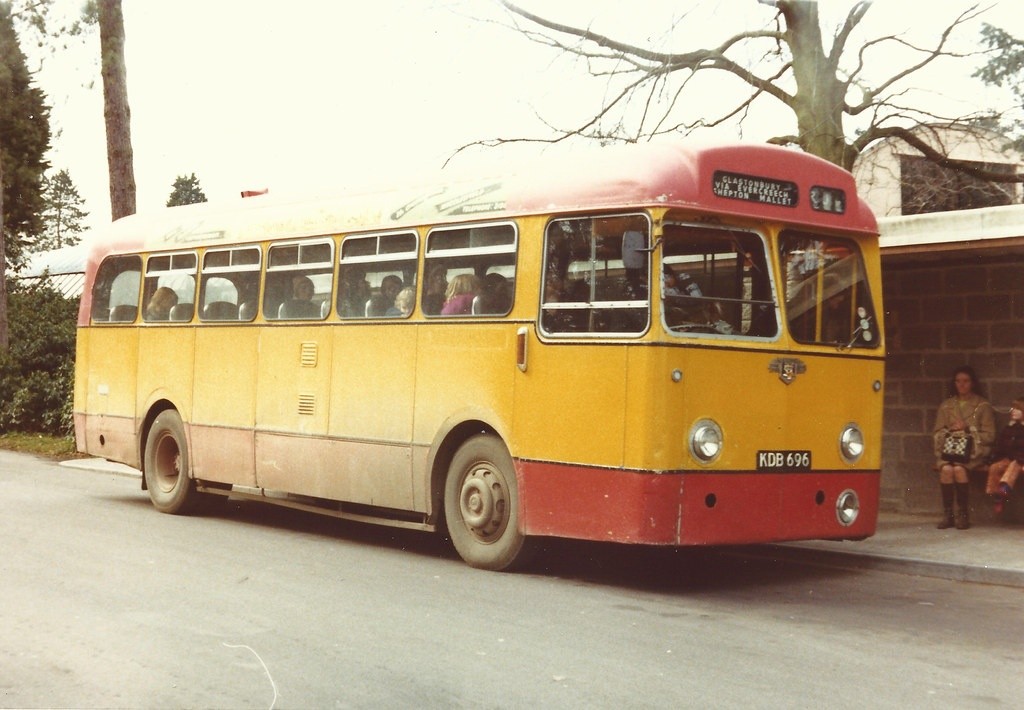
[471,295,482,315]
[320,297,353,319]
[109,305,138,322]
[239,300,268,320]
[203,300,239,320]
[365,299,378,317]
[278,303,289,318]
[169,302,194,322]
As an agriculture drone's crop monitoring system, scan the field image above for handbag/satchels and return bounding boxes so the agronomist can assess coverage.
[939,426,973,462]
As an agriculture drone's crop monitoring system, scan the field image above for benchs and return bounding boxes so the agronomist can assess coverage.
[933,463,1024,478]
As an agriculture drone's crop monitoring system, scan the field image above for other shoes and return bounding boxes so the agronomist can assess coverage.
[979,490,1007,523]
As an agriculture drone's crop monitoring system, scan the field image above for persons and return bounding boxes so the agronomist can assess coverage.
[292,277,314,300]
[987,398,1024,512]
[379,274,418,318]
[145,286,177,322]
[439,272,508,316]
[932,365,995,530]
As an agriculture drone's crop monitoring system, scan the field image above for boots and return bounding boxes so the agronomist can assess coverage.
[937,483,955,530]
[955,482,971,529]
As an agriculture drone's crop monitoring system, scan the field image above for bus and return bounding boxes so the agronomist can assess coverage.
[74,138,891,571]
